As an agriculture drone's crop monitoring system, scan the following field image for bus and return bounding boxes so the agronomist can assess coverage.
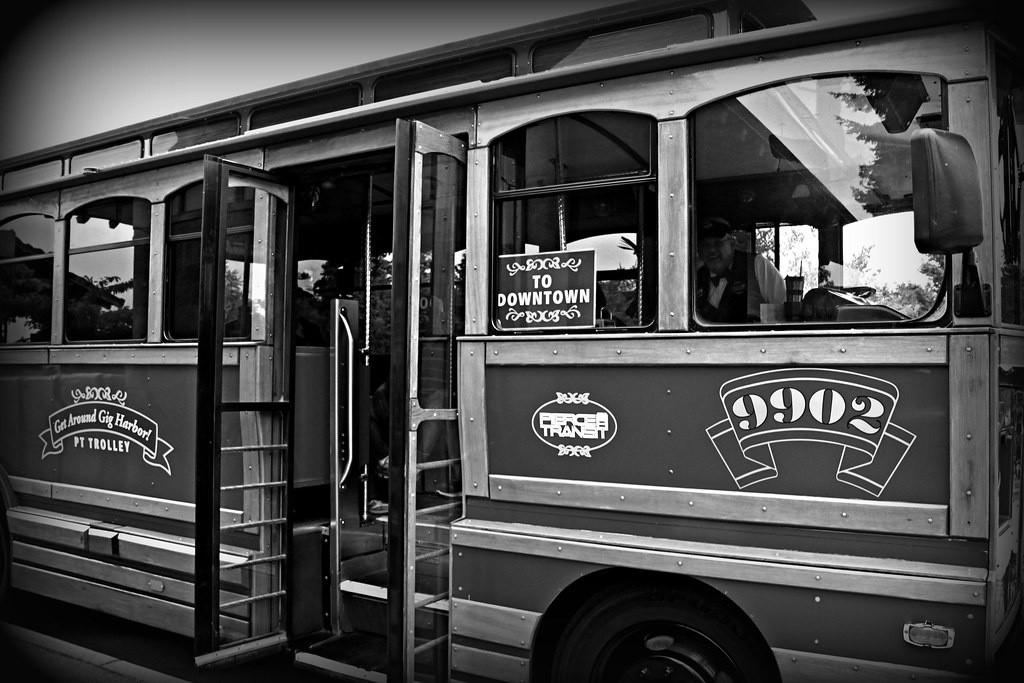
[0,0,1023,683]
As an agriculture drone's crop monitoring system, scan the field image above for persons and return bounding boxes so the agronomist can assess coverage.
[295,288,463,514]
[170,283,199,337]
[696,217,787,323]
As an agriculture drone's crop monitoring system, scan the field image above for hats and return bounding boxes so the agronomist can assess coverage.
[699,217,731,241]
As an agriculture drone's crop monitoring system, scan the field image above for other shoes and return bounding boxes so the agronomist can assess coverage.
[367,500,388,515]
[376,454,391,478]
